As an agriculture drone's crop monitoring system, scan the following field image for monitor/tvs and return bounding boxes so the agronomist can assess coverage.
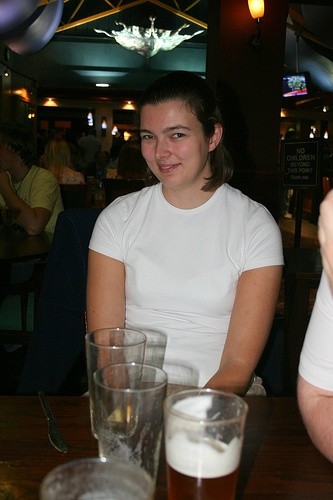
[279,72,312,101]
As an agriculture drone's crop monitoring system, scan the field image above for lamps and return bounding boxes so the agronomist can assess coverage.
[247,0,265,50]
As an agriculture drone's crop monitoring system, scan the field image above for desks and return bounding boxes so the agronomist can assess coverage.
[0,393,333,500]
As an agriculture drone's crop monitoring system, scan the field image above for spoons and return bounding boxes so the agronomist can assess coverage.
[38,391,72,454]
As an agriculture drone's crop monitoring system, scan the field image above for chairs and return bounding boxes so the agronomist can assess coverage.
[0,176,143,343]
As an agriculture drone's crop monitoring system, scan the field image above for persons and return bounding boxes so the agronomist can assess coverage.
[0,125,63,236]
[298,189,333,467]
[85,72,285,398]
[44,128,102,184]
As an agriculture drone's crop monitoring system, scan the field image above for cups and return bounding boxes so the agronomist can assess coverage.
[93,364,168,487]
[40,458,155,500]
[84,328,147,437]
[164,389,250,500]
